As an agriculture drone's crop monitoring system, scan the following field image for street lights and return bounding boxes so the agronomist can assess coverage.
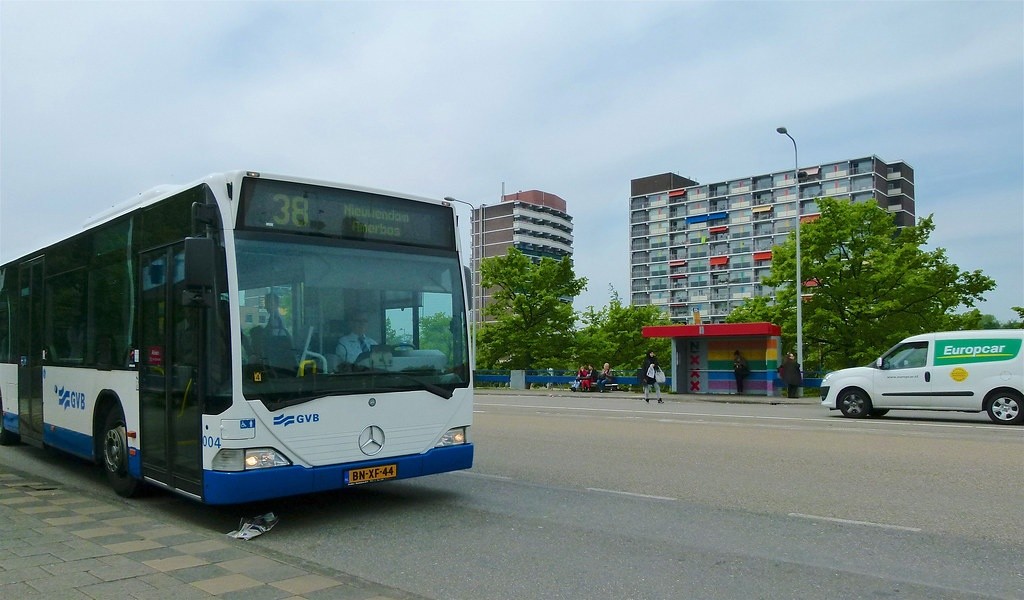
[444,196,476,388]
[776,127,803,396]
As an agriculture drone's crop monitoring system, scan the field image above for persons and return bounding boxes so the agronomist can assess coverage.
[262,293,284,329]
[170,305,195,365]
[783,353,802,399]
[641,349,665,405]
[334,309,379,362]
[733,349,747,395]
[569,362,613,393]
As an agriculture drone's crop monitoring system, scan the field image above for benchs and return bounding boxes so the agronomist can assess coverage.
[569,381,620,393]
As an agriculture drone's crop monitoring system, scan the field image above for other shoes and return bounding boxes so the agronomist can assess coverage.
[570,387,576,392]
[735,392,744,395]
[658,398,664,404]
[582,388,584,391]
[587,389,590,391]
[646,399,649,403]
[600,390,603,393]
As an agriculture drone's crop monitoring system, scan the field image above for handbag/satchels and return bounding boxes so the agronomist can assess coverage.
[742,367,750,378]
[779,367,785,377]
[654,366,666,383]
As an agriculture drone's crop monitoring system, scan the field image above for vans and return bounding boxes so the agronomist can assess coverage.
[819,328,1024,426]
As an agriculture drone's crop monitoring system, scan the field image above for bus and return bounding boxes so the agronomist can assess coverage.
[0,169,474,510]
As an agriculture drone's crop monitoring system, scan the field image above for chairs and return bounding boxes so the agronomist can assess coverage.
[322,320,347,356]
[260,325,304,381]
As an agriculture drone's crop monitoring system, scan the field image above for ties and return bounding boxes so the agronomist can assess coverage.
[358,337,369,353]
